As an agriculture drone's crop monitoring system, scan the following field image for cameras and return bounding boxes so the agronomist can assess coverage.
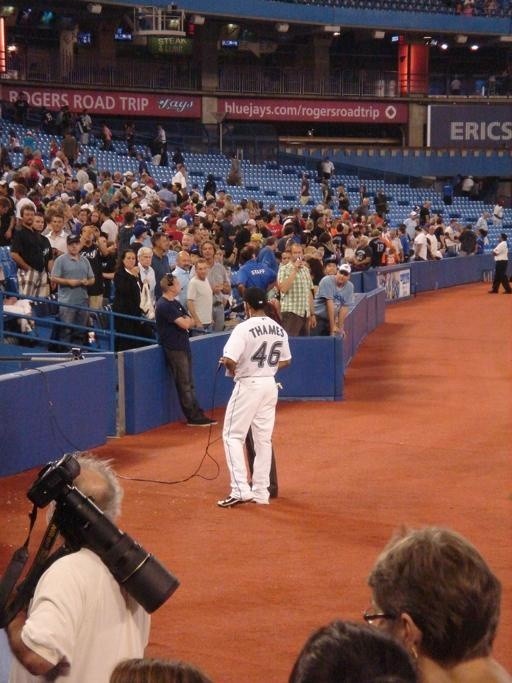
[27,451,180,615]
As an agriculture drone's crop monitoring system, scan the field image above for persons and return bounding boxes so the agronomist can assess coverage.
[289,618,418,683]
[0,92,512,352]
[246,301,282,498]
[4,452,151,683]
[155,273,218,427]
[363,528,511,683]
[216,287,292,507]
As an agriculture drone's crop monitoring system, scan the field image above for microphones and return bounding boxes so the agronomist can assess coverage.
[215,362,222,374]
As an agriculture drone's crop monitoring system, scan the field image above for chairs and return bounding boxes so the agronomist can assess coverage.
[0,122,512,349]
[284,0,509,20]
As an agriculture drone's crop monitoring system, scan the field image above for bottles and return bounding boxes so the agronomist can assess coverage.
[296,257,303,269]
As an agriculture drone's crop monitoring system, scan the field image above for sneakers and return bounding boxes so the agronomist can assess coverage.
[501,290,512,293]
[186,415,218,426]
[487,288,498,293]
[217,496,254,506]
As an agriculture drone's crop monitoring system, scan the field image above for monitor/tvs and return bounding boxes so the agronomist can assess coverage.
[114,26,132,41]
[77,31,94,49]
[217,39,239,49]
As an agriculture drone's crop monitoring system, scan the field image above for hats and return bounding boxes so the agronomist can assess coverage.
[339,263,352,273]
[133,218,153,235]
[323,254,338,263]
[67,234,81,243]
[242,286,266,305]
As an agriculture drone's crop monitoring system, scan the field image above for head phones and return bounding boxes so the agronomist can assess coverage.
[167,272,173,286]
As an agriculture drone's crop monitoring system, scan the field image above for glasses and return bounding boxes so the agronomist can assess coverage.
[361,603,400,630]
